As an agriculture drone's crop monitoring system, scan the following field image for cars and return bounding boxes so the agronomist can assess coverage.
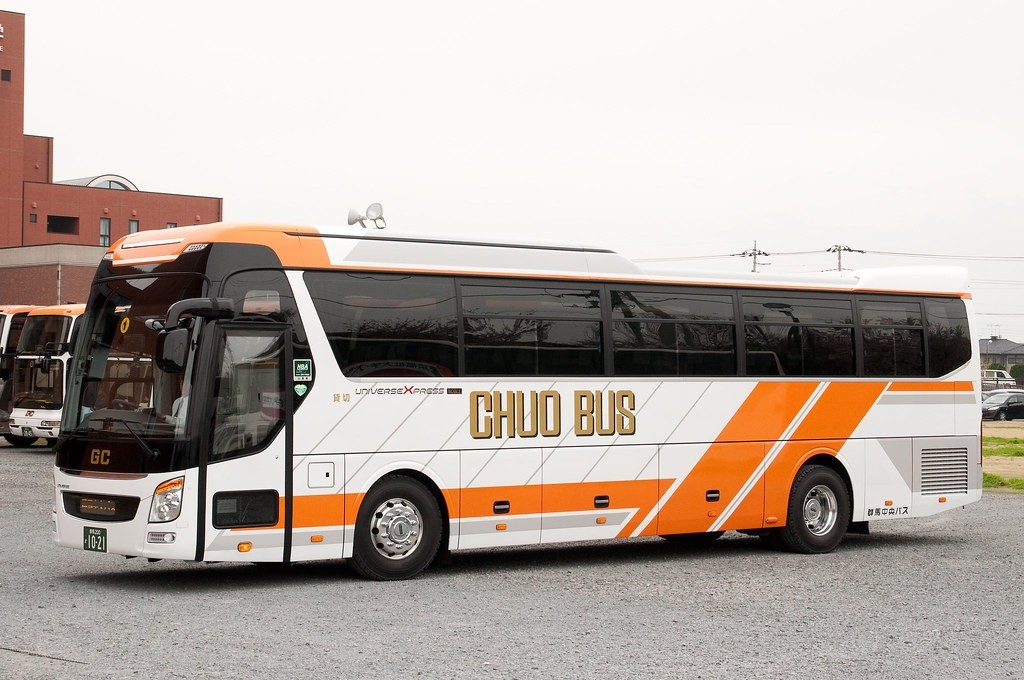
[982,393,1024,422]
[980,388,1024,404]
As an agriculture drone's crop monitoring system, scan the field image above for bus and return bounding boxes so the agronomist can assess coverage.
[50,224,986,586]
[0,304,50,449]
[9,306,194,447]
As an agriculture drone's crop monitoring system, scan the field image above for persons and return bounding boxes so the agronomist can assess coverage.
[157,377,192,435]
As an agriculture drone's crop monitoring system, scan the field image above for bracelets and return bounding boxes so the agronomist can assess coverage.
[163,416,166,420]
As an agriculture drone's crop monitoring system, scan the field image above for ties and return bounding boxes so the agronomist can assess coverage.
[174,398,183,419]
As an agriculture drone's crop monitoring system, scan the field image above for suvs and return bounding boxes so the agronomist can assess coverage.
[981,370,1017,389]
